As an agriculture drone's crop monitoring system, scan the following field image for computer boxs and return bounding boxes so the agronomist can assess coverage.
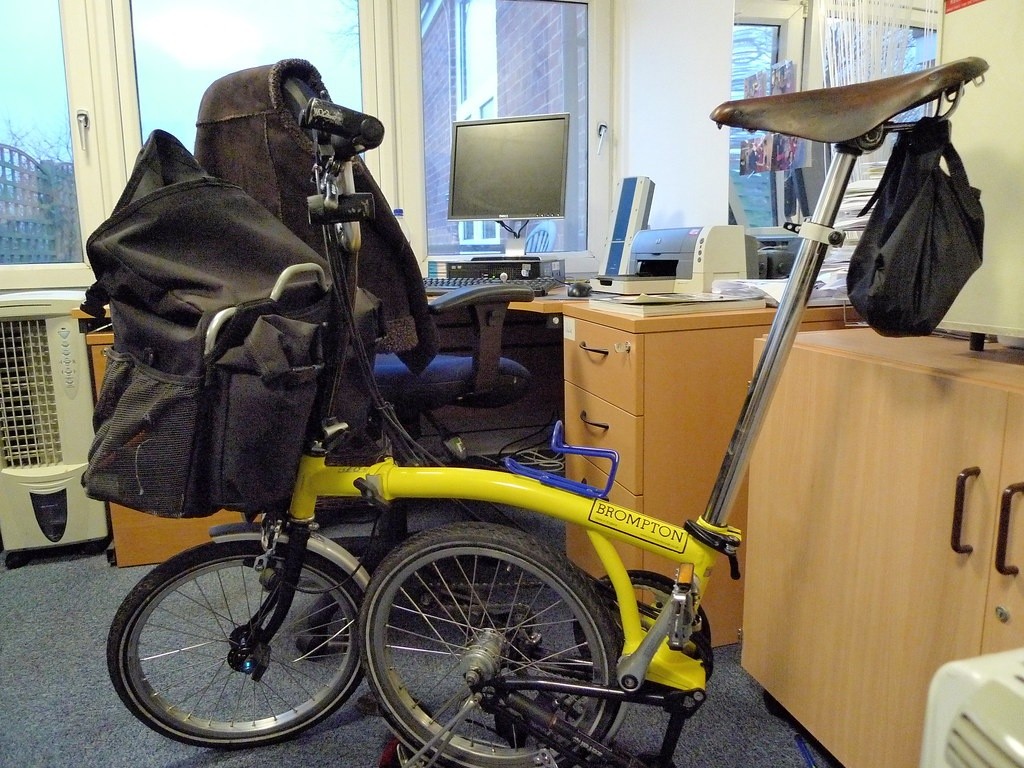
[446,259,566,288]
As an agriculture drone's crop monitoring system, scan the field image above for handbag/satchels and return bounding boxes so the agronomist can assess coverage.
[78,127,332,326]
[847,114,987,339]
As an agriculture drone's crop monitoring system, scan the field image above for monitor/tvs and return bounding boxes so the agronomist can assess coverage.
[448,112,571,260]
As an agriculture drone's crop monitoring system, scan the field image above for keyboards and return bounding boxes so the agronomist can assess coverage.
[422,278,556,296]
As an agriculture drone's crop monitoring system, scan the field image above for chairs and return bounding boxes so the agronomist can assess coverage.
[195,59,535,502]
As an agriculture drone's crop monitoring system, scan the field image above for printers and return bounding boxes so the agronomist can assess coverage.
[591,225,748,297]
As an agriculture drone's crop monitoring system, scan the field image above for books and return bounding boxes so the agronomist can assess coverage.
[589,292,767,319]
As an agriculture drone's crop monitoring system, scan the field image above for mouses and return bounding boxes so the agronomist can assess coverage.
[567,282,592,297]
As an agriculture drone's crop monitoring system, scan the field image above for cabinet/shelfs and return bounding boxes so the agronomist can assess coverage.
[72,305,262,568]
[740,329,1024,768]
[560,299,862,649]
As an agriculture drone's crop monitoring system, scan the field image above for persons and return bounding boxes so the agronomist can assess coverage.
[740,70,799,175]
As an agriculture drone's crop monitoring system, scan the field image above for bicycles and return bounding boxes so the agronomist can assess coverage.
[79,55,990,768]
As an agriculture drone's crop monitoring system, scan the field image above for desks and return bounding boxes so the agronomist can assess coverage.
[426,282,581,315]
